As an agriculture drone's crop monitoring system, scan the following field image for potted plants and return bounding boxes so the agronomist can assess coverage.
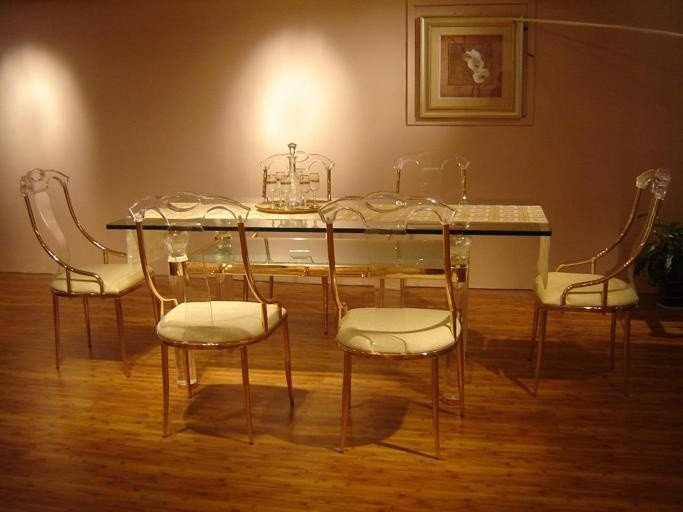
[633,212,683,310]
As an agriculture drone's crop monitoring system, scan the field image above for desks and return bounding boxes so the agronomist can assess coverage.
[112,197,554,401]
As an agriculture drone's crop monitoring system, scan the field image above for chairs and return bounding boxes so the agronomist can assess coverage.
[530,167,670,398]
[247,143,337,337]
[17,168,160,376]
[319,195,471,455]
[129,192,299,444]
[372,155,473,320]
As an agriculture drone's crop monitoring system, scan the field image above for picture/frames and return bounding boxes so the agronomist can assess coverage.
[406,1,533,127]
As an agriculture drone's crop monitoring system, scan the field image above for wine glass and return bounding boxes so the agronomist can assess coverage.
[269,167,330,210]
